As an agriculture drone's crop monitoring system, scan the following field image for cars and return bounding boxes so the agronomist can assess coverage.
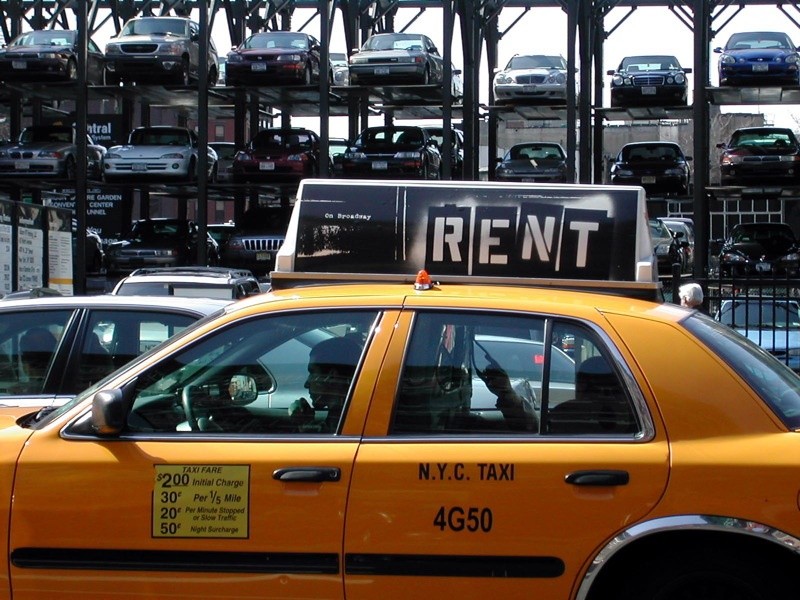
[718,222,800,281]
[712,293,800,374]
[648,216,697,275]
[68,217,104,276]
[0,28,106,84]
[607,55,692,108]
[493,141,568,183]
[465,332,582,420]
[103,124,464,185]
[104,17,348,89]
[0,181,799,600]
[0,295,540,443]
[0,122,107,187]
[607,140,694,196]
[493,53,578,100]
[716,126,800,188]
[713,31,800,91]
[104,217,218,282]
[348,33,463,103]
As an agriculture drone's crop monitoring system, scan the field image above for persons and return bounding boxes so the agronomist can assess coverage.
[478,355,627,434]
[666,238,800,306]
[0,327,59,396]
[678,283,703,309]
[288,337,363,436]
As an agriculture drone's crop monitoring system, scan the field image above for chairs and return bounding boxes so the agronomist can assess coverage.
[774,140,787,146]
[631,155,643,161]
[546,154,557,158]
[79,332,115,387]
[661,64,669,70]
[519,154,529,158]
[736,44,751,49]
[628,66,639,71]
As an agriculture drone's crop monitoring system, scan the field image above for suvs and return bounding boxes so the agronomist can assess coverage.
[217,205,294,272]
[106,266,273,356]
